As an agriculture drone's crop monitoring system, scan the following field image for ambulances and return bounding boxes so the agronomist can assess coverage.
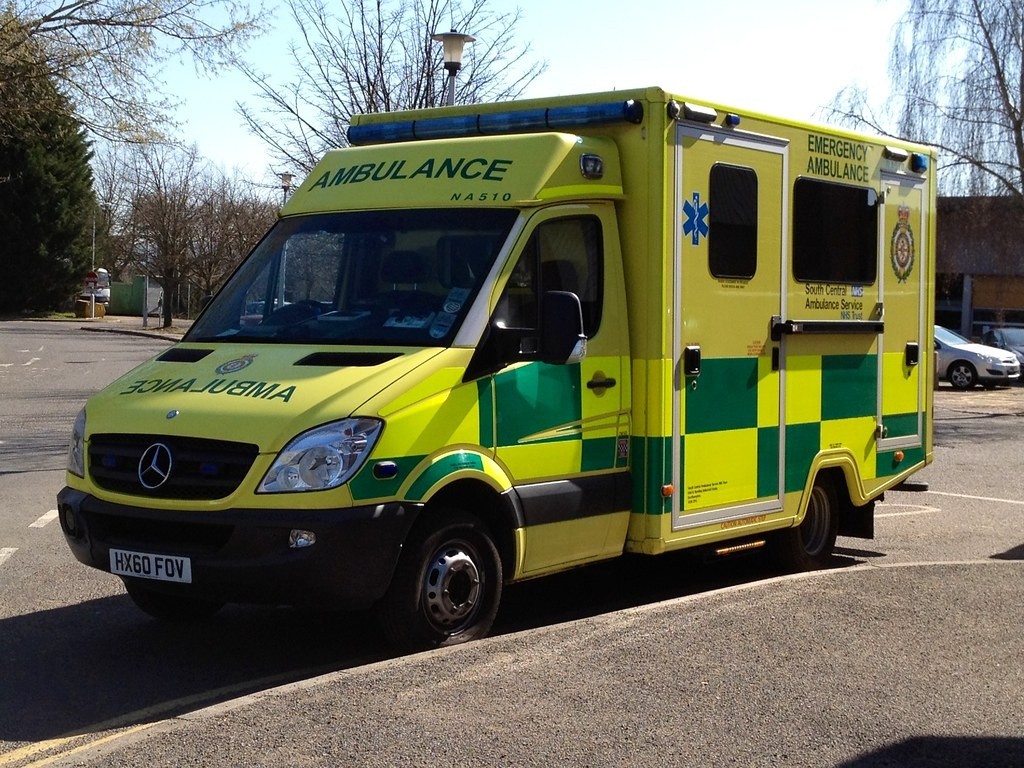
[56,86,939,649]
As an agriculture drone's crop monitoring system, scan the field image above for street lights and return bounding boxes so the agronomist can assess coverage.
[275,171,296,309]
[430,29,476,106]
[91,202,112,318]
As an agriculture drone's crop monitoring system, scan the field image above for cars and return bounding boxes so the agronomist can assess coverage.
[980,328,1024,380]
[933,325,1021,391]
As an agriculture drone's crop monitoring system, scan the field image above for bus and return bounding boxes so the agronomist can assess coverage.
[75,268,111,308]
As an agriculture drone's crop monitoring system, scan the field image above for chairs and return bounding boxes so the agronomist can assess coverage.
[516,259,593,329]
[372,251,444,309]
